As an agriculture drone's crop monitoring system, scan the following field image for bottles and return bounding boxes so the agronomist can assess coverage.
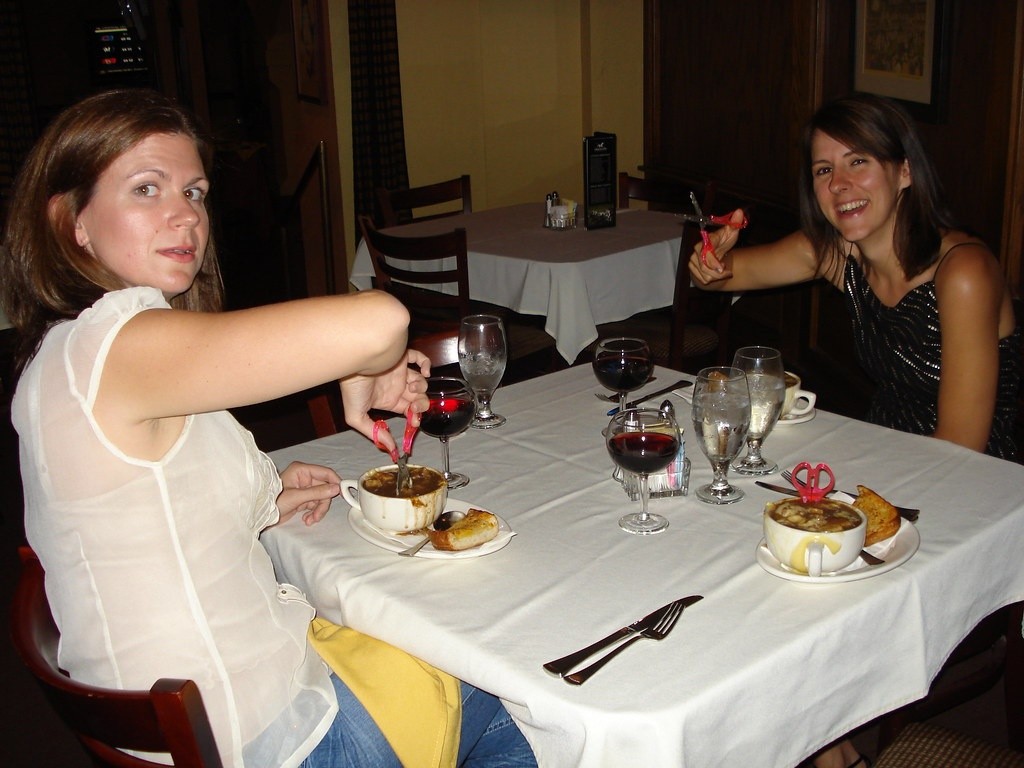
[612,410,639,482]
[544,192,562,226]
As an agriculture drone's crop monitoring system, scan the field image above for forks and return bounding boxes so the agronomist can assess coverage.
[595,377,657,402]
[566,602,685,685]
[781,471,921,515]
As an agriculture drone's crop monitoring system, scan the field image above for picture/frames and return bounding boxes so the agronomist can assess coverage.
[288,0,330,107]
[847,0,955,127]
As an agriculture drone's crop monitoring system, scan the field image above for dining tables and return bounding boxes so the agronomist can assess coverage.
[258,362,1024,768]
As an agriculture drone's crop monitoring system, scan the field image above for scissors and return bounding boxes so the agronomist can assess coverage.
[673,191,749,271]
[791,463,835,506]
[374,402,423,493]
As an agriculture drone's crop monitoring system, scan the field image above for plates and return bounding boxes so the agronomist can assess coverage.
[774,398,816,426]
[757,516,919,582]
[348,498,511,559]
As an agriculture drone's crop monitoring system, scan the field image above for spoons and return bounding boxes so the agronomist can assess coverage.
[398,511,465,556]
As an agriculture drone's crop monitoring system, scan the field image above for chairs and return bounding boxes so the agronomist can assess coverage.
[876,300,1024,757]
[303,309,509,437]
[379,173,472,229]
[868,719,1024,768]
[618,171,720,216]
[6,538,223,768]
[582,190,745,373]
[356,214,554,386]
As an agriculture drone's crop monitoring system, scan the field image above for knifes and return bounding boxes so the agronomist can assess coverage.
[608,379,693,417]
[543,595,704,678]
[755,481,919,523]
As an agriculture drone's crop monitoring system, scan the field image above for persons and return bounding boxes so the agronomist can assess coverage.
[0,89,539,768]
[689,90,1024,768]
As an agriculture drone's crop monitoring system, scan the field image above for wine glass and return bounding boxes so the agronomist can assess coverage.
[690,366,752,505]
[459,315,507,431]
[729,345,783,475]
[420,377,477,489]
[605,407,680,535]
[592,336,652,437]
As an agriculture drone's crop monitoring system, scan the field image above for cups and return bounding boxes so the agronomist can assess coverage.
[339,464,447,532]
[739,372,814,417]
[765,497,868,577]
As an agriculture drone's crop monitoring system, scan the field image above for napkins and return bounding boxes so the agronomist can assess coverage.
[363,519,517,559]
[829,489,910,572]
[672,384,696,405]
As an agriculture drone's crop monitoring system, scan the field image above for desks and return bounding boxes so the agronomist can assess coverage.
[348,201,696,366]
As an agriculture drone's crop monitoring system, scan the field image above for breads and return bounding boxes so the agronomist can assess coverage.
[704,371,730,393]
[427,508,499,552]
[852,485,901,548]
